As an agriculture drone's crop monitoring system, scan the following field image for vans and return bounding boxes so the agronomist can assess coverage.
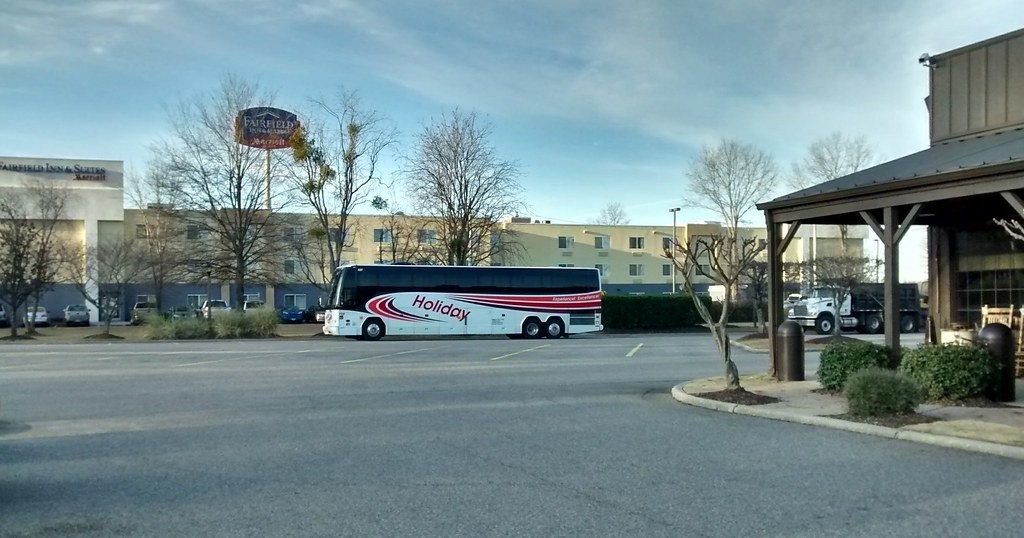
[242,299,266,312]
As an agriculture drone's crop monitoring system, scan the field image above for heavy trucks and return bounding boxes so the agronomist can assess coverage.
[787,277,922,337]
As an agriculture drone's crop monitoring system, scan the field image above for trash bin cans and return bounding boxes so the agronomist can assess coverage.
[777,321,806,381]
[978,323,1016,402]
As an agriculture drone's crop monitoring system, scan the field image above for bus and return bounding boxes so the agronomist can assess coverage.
[318,261,605,342]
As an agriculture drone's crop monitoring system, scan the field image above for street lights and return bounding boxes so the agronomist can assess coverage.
[873,238,880,284]
[669,206,681,295]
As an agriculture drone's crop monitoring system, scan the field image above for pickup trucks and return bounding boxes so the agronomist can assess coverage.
[128,302,160,325]
[200,299,232,319]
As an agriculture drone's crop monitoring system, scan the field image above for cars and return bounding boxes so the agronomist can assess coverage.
[303,305,326,323]
[62,305,91,326]
[0,303,11,327]
[163,305,198,320]
[274,305,305,323]
[22,307,51,327]
